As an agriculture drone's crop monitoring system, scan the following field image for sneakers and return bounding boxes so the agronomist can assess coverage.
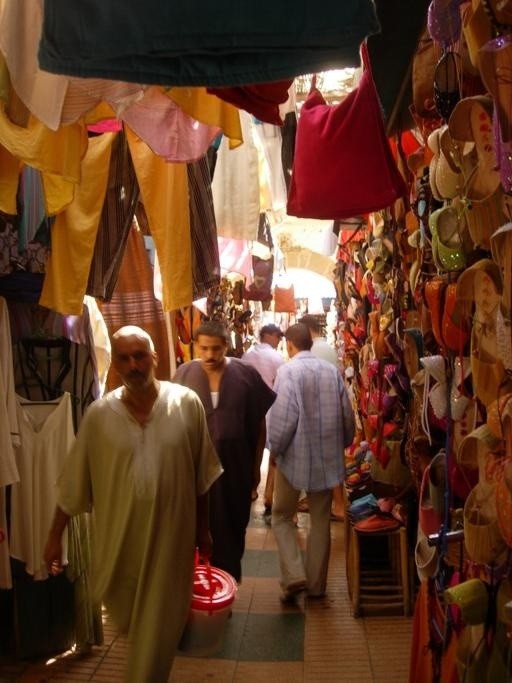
[332,189,424,471]
[279,581,310,603]
[331,513,345,523]
[426,125,463,203]
[414,521,439,582]
[424,280,471,351]
[344,461,407,533]
[477,35,512,193]
[419,453,454,540]
[428,206,466,272]
[434,52,462,120]
[419,355,447,420]
[455,259,508,409]
[456,424,507,563]
[449,355,473,421]
[304,592,330,603]
[461,1,491,67]
[448,95,508,250]
[490,222,512,375]
[443,578,512,682]
[487,393,512,551]
[175,281,254,368]
[264,507,271,525]
[298,497,310,512]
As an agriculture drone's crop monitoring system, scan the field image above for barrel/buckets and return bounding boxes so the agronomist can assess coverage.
[173,546,241,658]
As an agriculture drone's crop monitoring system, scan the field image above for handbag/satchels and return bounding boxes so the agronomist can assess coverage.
[285,37,408,219]
[243,213,274,301]
[275,258,298,314]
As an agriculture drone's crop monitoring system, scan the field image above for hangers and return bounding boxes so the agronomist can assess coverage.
[11,356,79,410]
[414,523,475,621]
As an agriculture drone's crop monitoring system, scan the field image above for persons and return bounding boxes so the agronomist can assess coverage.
[44,324,226,681]
[295,315,348,521]
[240,326,286,520]
[169,322,281,620]
[265,323,356,604]
